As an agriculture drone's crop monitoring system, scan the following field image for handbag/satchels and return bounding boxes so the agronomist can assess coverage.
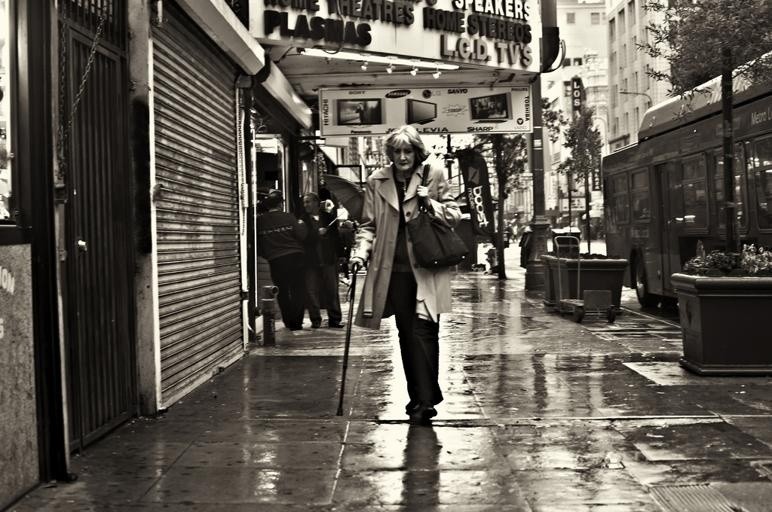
[406,210,469,269]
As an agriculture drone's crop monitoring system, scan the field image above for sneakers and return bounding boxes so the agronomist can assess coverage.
[312,322,343,329]
[406,399,438,420]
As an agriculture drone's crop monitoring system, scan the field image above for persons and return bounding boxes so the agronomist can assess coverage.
[298,193,345,329]
[350,125,463,419]
[475,100,507,119]
[339,213,358,280]
[258,190,309,331]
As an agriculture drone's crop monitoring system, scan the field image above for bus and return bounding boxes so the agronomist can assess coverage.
[599,51,771,309]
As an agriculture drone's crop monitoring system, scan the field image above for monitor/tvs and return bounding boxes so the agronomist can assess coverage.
[333,98,386,133]
[404,99,437,126]
[468,92,513,124]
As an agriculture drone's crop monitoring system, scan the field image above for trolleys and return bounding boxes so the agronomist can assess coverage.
[554,235,622,323]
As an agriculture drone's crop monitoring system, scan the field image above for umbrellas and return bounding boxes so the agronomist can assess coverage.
[321,172,367,228]
